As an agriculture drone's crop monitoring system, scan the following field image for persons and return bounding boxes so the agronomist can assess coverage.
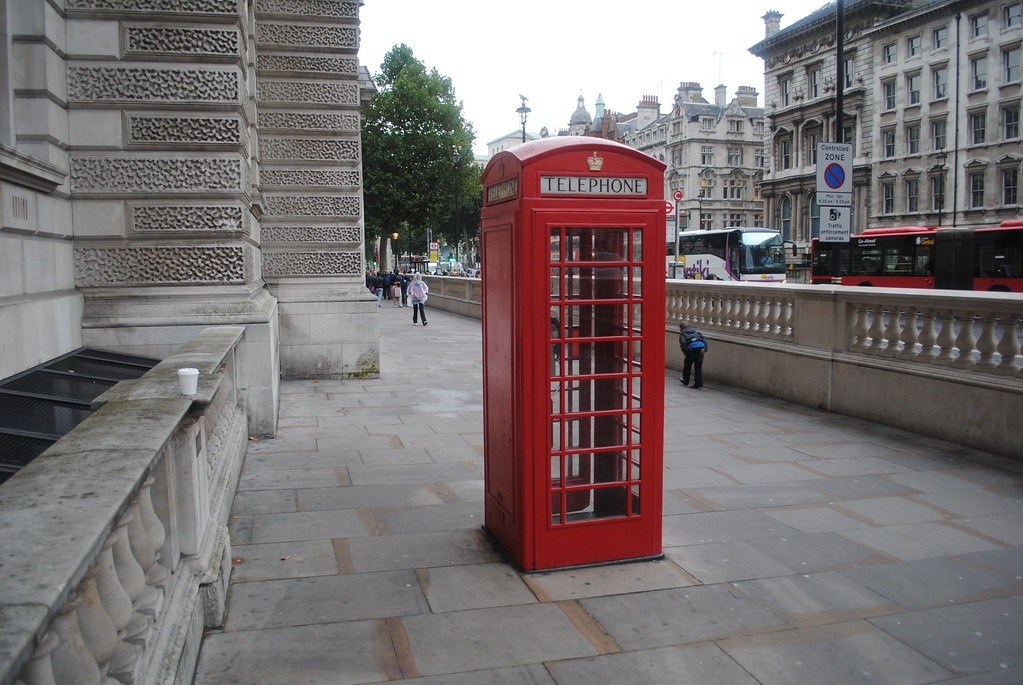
[366,270,411,308]
[408,268,464,277]
[684,272,723,280]
[679,322,707,388]
[406,272,428,326]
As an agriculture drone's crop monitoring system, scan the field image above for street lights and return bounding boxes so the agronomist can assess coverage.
[516,95,531,140]
[449,144,463,264]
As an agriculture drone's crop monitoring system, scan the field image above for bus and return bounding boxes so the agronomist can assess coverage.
[809,218,1023,293]
[662,225,798,283]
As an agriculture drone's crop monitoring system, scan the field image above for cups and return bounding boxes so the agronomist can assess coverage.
[176,368,199,395]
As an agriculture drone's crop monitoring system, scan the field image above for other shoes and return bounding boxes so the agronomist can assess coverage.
[690,385,703,389]
[424,321,428,326]
[414,323,417,325]
[680,378,689,385]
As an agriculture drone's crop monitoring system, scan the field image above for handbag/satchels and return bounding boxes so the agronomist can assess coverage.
[395,287,401,297]
